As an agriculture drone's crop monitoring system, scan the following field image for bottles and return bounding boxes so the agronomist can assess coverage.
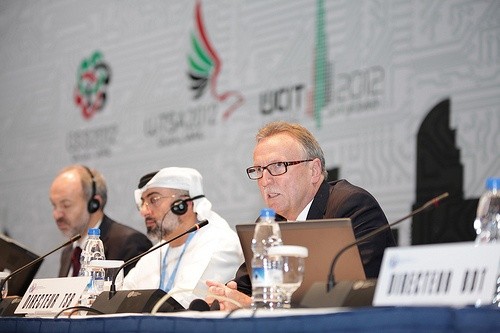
[251,209,284,310]
[474,176,500,243]
[78,228,106,315]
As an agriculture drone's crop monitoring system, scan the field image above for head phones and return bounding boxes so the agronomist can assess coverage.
[170,194,206,215]
[84,166,100,213]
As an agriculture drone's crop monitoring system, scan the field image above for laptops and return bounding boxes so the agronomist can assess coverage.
[235,217,367,307]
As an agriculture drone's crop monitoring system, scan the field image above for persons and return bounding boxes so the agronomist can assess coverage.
[51,165,152,280]
[206,121,395,310]
[125,167,245,309]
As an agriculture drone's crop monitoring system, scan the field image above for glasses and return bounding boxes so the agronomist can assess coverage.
[137,193,188,212]
[247,159,313,180]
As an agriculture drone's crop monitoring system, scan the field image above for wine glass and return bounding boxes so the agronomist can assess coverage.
[265,245,309,309]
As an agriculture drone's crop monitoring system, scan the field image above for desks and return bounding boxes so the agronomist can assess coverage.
[0,309,500,333]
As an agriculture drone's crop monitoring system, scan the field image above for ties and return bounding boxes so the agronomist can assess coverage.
[71,246,82,277]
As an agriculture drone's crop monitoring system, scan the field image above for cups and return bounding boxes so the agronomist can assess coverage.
[91,261,124,293]
[0,273,8,298]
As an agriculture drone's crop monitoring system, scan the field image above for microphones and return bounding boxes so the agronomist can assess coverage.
[87,220,209,315]
[0,234,81,317]
[302,192,449,308]
